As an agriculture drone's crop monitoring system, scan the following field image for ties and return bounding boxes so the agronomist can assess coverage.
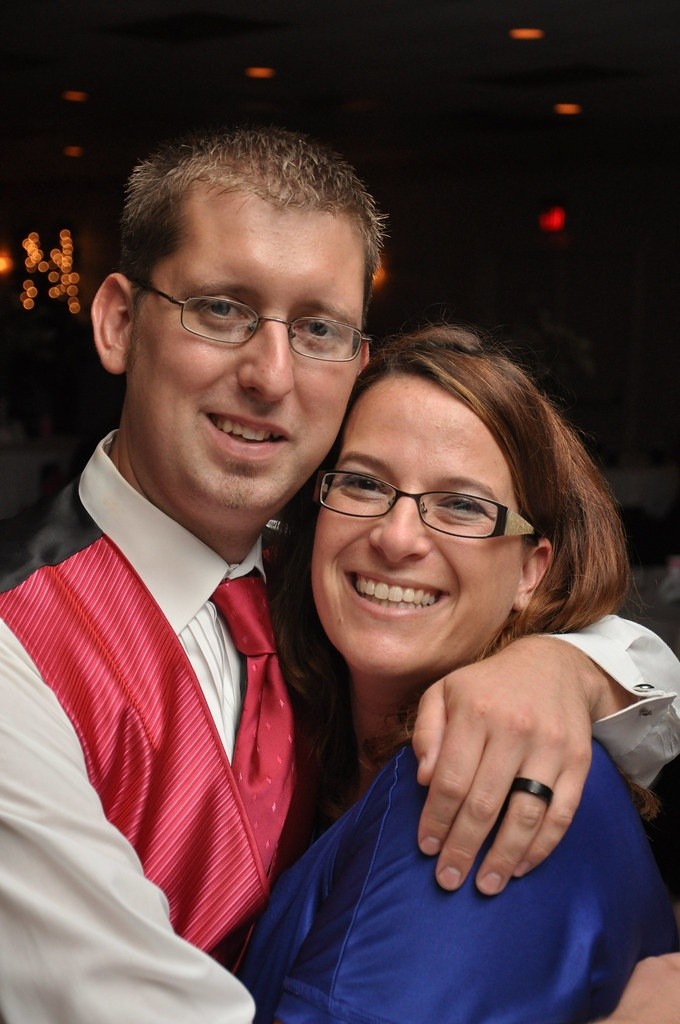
[211,573,295,877]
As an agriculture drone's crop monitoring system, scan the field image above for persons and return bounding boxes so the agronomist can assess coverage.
[1,125,680,1024]
[236,327,680,1024]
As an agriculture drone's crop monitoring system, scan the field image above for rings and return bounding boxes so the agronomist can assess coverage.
[513,776,553,804]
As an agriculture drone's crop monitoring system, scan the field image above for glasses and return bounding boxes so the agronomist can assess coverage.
[135,281,373,363]
[311,470,547,539]
